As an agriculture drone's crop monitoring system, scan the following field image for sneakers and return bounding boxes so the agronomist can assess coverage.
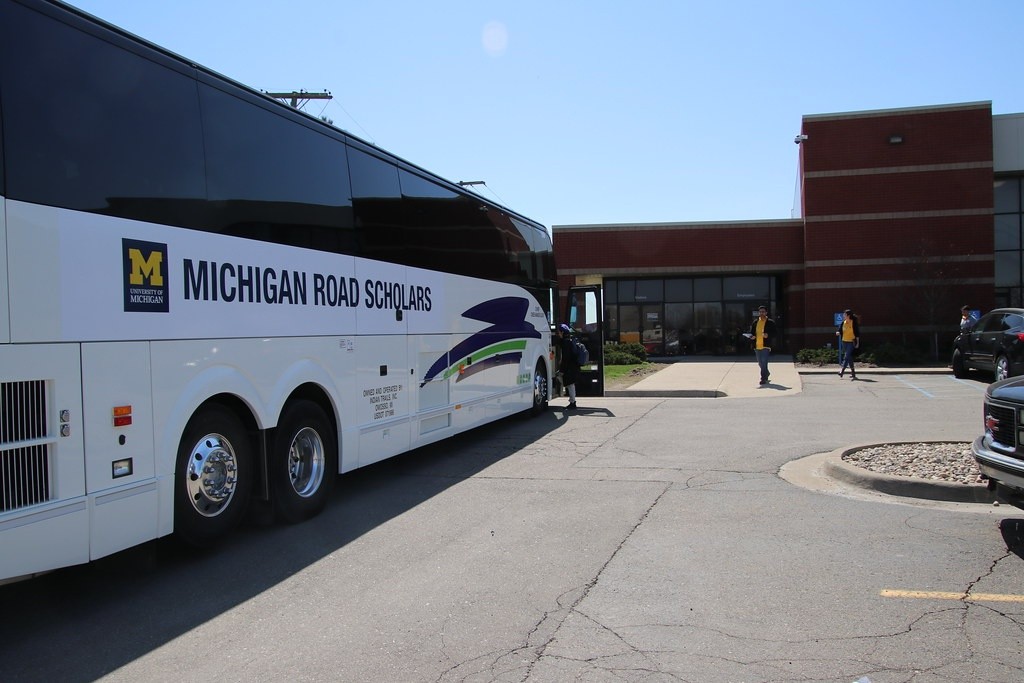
[760,377,767,384]
[766,370,770,380]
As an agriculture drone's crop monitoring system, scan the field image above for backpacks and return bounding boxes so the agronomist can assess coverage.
[565,336,589,365]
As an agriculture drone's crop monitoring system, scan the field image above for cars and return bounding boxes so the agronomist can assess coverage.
[971,375,1024,488]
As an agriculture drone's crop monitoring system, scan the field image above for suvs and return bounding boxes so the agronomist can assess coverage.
[952,307,1024,382]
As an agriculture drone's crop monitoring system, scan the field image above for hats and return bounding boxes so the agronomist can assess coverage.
[559,324,569,332]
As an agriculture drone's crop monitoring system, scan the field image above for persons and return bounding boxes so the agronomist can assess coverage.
[557,324,581,410]
[960,305,976,334]
[750,306,774,384]
[836,310,860,378]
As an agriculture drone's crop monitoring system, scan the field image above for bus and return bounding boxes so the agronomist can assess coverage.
[0,1,604,582]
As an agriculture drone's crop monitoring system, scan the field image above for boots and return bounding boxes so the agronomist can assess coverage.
[837,367,846,377]
[849,368,855,378]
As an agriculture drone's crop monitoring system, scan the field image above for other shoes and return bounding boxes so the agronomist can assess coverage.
[947,364,954,368]
[564,402,577,410]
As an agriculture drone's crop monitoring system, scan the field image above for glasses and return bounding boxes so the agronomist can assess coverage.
[843,313,849,315]
[758,311,767,313]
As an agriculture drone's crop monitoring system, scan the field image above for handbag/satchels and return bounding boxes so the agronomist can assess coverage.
[853,339,858,347]
